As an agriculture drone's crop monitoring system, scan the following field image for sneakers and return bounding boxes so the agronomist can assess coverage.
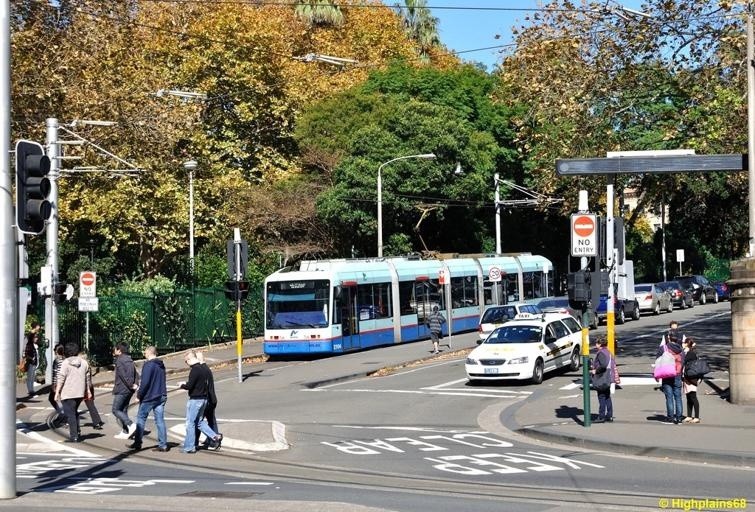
[432,350,440,355]
[592,416,614,423]
[662,416,686,424]
[689,417,700,424]
[113,422,137,440]
[93,423,104,430]
[681,416,692,424]
[179,433,223,454]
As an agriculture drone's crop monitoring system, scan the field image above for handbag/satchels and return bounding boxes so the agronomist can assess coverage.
[684,357,712,379]
[18,357,28,372]
[590,367,613,391]
[653,343,678,379]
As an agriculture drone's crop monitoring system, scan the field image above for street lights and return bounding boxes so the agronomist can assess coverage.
[375,153,437,258]
[182,159,197,275]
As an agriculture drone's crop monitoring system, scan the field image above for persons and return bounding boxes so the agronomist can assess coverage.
[661,320,687,344]
[681,337,702,424]
[589,334,616,423]
[426,305,447,354]
[77,347,106,430]
[194,352,222,451]
[124,345,171,451]
[110,340,139,440]
[24,330,38,396]
[52,341,92,443]
[29,322,43,387]
[655,334,684,423]
[176,351,223,455]
[48,343,66,419]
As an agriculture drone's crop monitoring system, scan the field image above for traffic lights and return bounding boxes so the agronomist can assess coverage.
[53,282,67,304]
[24,153,51,223]
[224,281,237,301]
[238,281,251,300]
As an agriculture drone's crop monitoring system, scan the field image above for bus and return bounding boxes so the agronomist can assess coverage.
[258,252,553,353]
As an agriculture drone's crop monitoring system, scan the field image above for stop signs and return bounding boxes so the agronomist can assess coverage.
[80,271,96,297]
[571,212,598,257]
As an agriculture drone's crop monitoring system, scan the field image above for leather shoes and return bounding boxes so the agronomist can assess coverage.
[63,435,81,443]
[124,443,142,449]
[151,447,169,453]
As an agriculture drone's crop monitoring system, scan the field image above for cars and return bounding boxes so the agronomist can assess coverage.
[463,311,582,385]
[477,272,729,344]
